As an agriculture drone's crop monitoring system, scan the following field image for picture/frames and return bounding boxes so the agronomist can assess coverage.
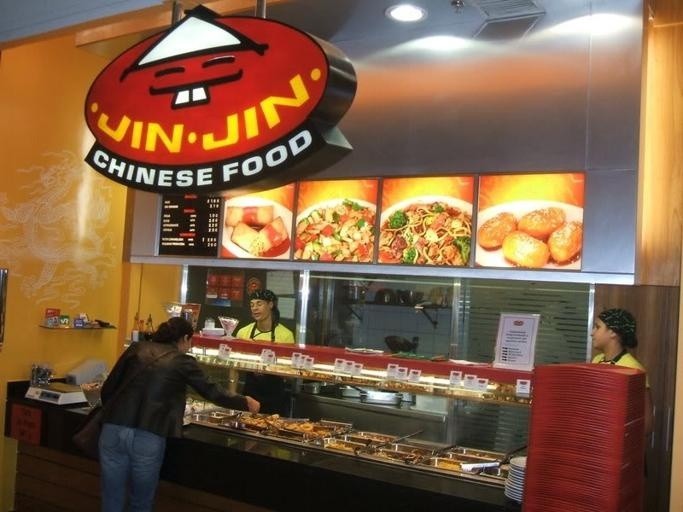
[493,311,542,372]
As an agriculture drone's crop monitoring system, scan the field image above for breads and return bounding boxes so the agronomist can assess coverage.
[478,207,583,268]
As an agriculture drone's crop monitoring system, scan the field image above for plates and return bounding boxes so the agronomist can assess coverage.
[503,456,527,504]
[221,196,293,260]
[476,201,583,273]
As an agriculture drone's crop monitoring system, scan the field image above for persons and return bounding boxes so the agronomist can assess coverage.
[97,316,260,511]
[330,305,367,348]
[588,306,653,484]
[228,287,295,416]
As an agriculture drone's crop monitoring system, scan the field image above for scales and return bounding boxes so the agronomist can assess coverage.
[24,382,88,406]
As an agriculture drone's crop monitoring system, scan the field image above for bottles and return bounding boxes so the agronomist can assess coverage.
[131,312,154,342]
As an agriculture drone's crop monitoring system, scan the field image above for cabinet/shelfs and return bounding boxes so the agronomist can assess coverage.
[129,337,535,510]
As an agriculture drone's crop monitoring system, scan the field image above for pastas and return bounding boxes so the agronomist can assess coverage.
[385,207,472,266]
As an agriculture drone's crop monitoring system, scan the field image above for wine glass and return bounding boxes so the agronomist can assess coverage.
[218,317,240,340]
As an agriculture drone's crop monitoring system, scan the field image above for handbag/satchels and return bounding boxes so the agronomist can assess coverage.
[70,404,105,464]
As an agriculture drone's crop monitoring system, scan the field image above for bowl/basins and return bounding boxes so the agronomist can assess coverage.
[373,288,424,307]
[203,328,225,337]
[385,335,419,354]
[303,382,320,394]
